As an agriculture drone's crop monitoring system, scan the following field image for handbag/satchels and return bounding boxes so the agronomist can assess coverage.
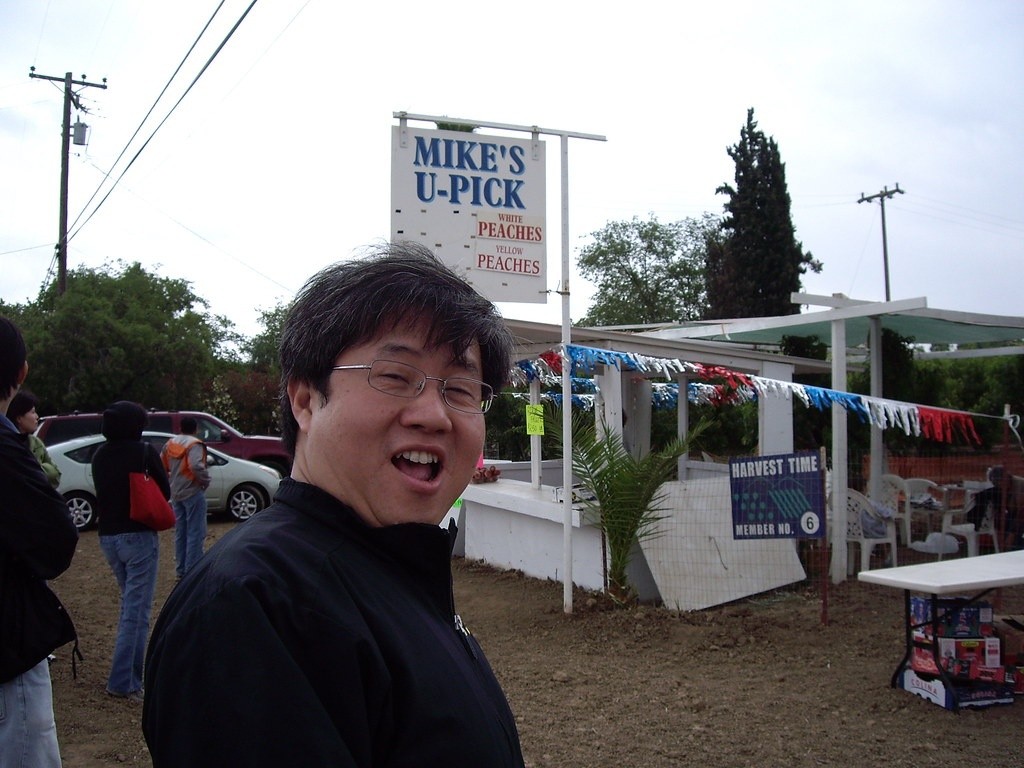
[129,441,177,532]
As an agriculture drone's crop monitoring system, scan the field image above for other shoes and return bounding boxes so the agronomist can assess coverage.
[108,686,145,702]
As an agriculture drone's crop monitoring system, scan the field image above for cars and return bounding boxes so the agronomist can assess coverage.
[43,428,284,535]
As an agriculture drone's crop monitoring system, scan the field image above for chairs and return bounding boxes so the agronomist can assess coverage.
[828,472,1001,577]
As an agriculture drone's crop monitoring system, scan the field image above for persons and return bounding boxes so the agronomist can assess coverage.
[849,475,895,573]
[159,414,212,583]
[141,242,529,768]
[6,389,62,662]
[602,401,627,427]
[990,466,1024,546]
[91,401,172,701]
[0,315,80,768]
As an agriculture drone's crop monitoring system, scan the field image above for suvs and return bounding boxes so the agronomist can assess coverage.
[32,408,294,482]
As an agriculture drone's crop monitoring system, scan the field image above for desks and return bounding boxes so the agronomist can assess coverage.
[858,548,1024,716]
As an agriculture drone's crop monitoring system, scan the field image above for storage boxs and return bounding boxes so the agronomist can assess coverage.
[552,483,597,504]
[903,593,1024,710]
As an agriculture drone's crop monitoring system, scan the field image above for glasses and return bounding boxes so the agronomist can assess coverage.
[332,359,499,415]
